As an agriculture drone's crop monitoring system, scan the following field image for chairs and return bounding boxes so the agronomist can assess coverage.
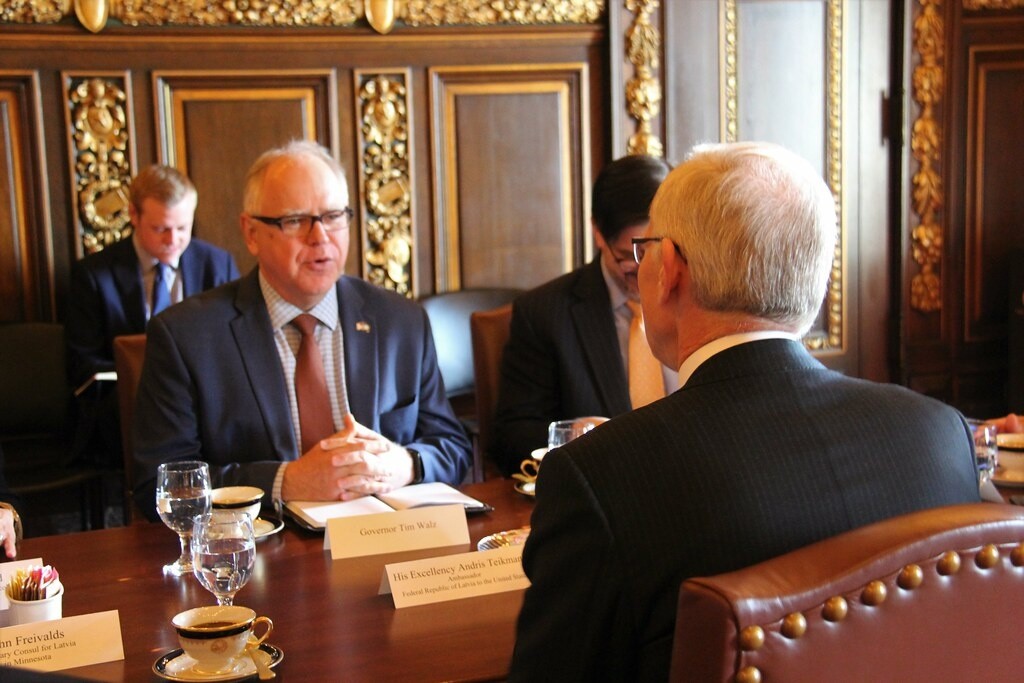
[1,288,1024,683]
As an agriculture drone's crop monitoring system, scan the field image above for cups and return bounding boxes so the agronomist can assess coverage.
[966,417,996,485]
[208,486,265,537]
[170,606,273,675]
[4,581,63,625]
[520,447,553,481]
[548,421,595,451]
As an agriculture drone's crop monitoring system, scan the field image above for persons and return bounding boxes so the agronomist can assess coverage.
[487,153,681,467]
[131,137,473,522]
[63,164,242,469]
[505,141,980,683]
[0,501,23,560]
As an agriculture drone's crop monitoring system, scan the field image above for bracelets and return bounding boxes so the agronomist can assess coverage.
[408,448,423,485]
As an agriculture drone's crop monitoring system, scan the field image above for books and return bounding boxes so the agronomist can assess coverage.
[274,481,495,541]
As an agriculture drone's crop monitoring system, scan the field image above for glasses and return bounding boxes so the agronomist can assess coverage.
[248,206,353,238]
[632,236,688,264]
[606,245,640,273]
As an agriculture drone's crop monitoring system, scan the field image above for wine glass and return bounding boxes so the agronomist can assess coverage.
[192,511,256,606]
[154,461,211,577]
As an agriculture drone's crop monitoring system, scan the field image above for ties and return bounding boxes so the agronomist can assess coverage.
[623,299,665,409]
[152,262,173,318]
[289,313,336,458]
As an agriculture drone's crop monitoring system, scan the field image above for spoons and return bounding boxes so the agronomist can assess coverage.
[248,633,276,680]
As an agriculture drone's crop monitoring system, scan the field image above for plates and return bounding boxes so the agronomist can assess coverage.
[979,433,1024,453]
[151,642,284,682]
[513,475,535,503]
[975,447,1024,486]
[208,516,284,542]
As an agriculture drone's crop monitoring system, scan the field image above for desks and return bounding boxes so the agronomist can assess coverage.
[0,472,1024,683]
